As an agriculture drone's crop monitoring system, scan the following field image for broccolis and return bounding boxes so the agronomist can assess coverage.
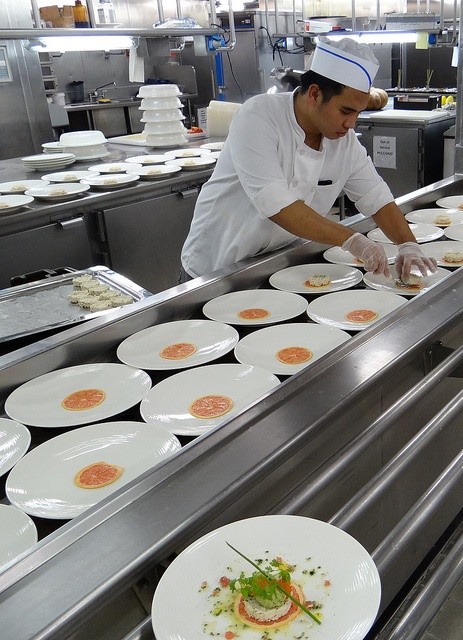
[335,118,455,213]
[0,184,229,296]
[201,10,305,103]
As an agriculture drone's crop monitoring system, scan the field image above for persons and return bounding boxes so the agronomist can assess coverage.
[179,35,438,285]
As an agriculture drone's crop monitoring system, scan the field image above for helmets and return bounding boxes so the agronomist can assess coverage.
[137,165,181,178]
[40,142,62,153]
[414,241,463,267]
[79,174,139,189]
[0,504,37,567]
[164,148,211,159]
[268,263,363,293]
[322,241,401,266]
[233,322,353,376]
[200,153,221,160]
[4,421,182,521]
[435,195,463,209]
[3,363,151,427]
[88,163,141,174]
[96,22,120,28]
[366,224,443,244]
[200,142,224,150]
[164,157,216,170]
[0,418,31,478]
[116,319,239,370]
[0,180,49,193]
[40,171,100,183]
[362,263,453,295]
[20,153,75,171]
[306,289,409,331]
[124,155,175,164]
[139,363,280,436]
[201,289,308,327]
[404,209,462,227]
[23,183,90,200]
[150,514,382,640]
[443,227,463,240]
[0,195,33,215]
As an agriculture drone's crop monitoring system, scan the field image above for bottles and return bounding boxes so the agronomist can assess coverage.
[310,32,380,94]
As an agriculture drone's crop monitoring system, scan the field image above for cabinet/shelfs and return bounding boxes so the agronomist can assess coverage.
[70,0,88,27]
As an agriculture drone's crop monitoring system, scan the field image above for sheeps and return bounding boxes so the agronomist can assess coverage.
[89,82,118,101]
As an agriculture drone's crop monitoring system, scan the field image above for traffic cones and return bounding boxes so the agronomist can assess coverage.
[366,87,388,110]
